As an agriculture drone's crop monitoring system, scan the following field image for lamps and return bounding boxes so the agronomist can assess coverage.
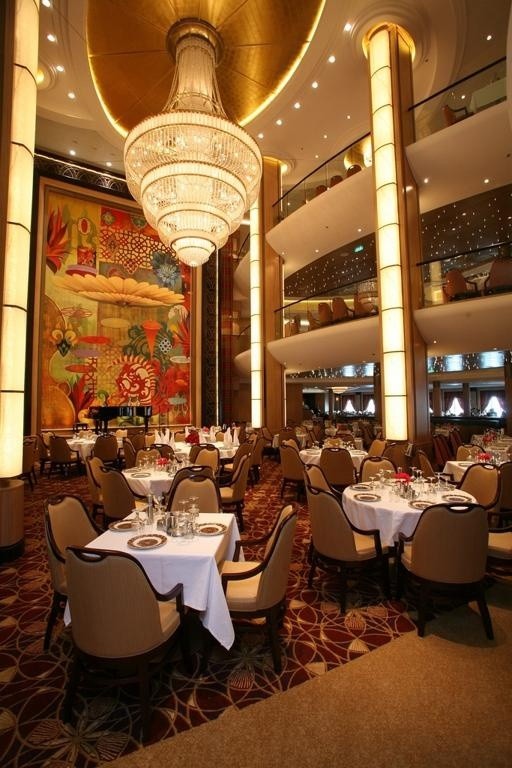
[124,14,261,269]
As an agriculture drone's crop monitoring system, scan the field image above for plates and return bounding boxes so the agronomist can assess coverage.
[108,520,146,531]
[129,534,167,550]
[196,522,227,536]
[442,494,472,505]
[355,494,380,502]
[350,482,372,490]
[409,500,435,511]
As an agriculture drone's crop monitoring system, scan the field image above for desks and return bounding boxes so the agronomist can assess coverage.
[471,75,508,114]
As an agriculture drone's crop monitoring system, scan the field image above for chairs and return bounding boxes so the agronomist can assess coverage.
[442,104,473,125]
[316,185,327,194]
[329,174,343,187]
[286,244,512,332]
[346,165,361,178]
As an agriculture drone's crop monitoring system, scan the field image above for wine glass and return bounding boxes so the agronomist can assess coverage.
[310,439,356,454]
[368,467,451,502]
[131,494,201,540]
[458,447,510,468]
[137,455,180,477]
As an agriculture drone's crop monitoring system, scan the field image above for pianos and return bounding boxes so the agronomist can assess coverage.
[93,406,152,435]
